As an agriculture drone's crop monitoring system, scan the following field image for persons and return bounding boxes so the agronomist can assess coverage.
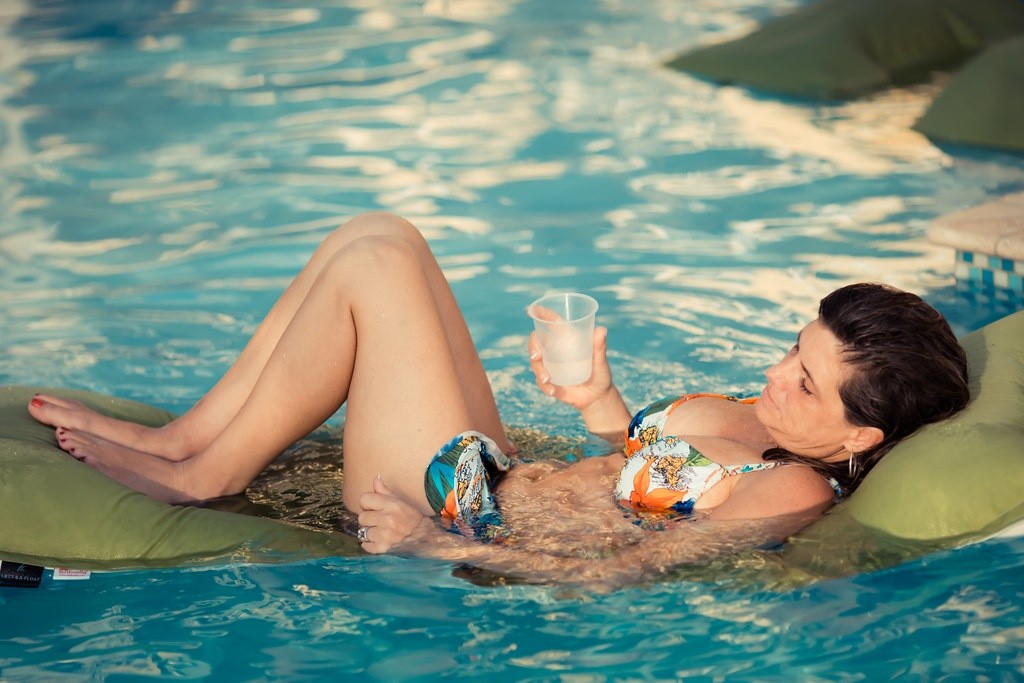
[27,212,971,588]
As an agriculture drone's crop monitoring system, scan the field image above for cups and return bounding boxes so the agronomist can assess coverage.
[527,291,599,389]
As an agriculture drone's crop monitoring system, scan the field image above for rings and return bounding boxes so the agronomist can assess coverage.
[357,526,372,542]
